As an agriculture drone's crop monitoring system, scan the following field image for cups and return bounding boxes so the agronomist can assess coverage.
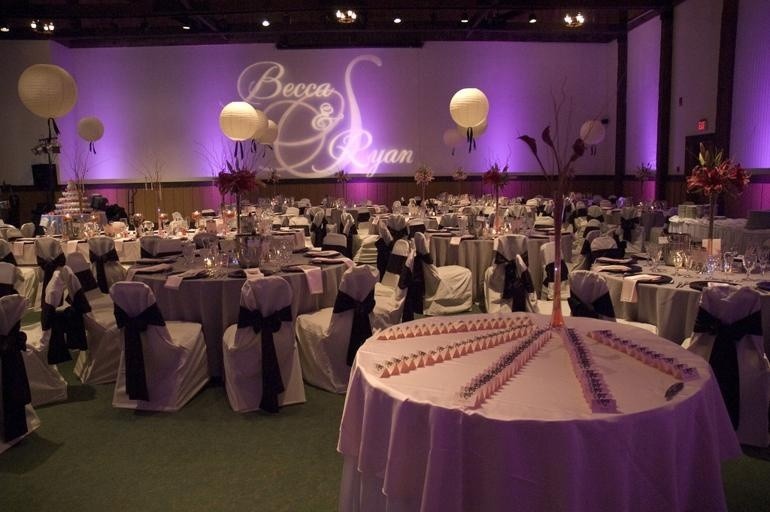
[182,236,295,278]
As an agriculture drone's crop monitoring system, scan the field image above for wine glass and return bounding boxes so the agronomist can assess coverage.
[646,242,769,282]
[458,218,466,236]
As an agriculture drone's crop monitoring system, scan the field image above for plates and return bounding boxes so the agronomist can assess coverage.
[137,257,177,264]
[534,227,555,231]
[165,270,209,280]
[281,246,344,272]
[129,264,173,273]
[427,227,482,240]
[596,256,770,292]
[529,233,549,238]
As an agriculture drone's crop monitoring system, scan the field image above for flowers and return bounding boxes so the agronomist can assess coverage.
[194,136,273,235]
[482,162,512,194]
[683,141,753,201]
[415,163,436,188]
[451,164,470,180]
[635,162,652,180]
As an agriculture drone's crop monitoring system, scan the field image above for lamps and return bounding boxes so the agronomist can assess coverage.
[28,16,66,36]
[335,8,357,23]
[558,10,585,30]
